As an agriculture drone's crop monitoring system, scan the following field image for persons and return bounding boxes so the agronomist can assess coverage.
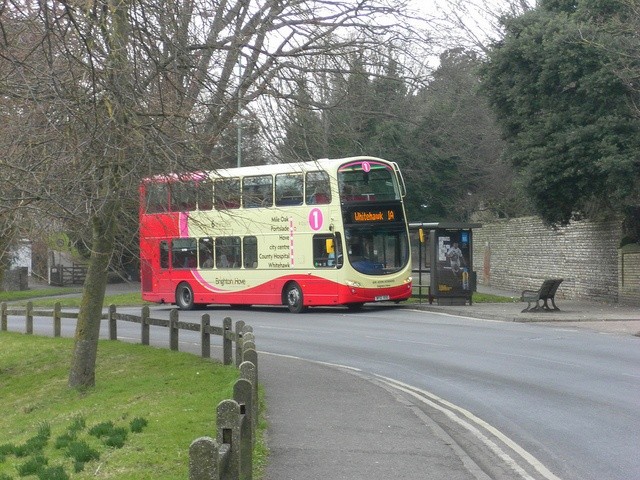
[446,241,466,268]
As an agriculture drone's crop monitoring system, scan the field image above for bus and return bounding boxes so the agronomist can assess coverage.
[138,154,413,313]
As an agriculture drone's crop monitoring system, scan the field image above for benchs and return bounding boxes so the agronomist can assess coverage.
[519,277,564,313]
